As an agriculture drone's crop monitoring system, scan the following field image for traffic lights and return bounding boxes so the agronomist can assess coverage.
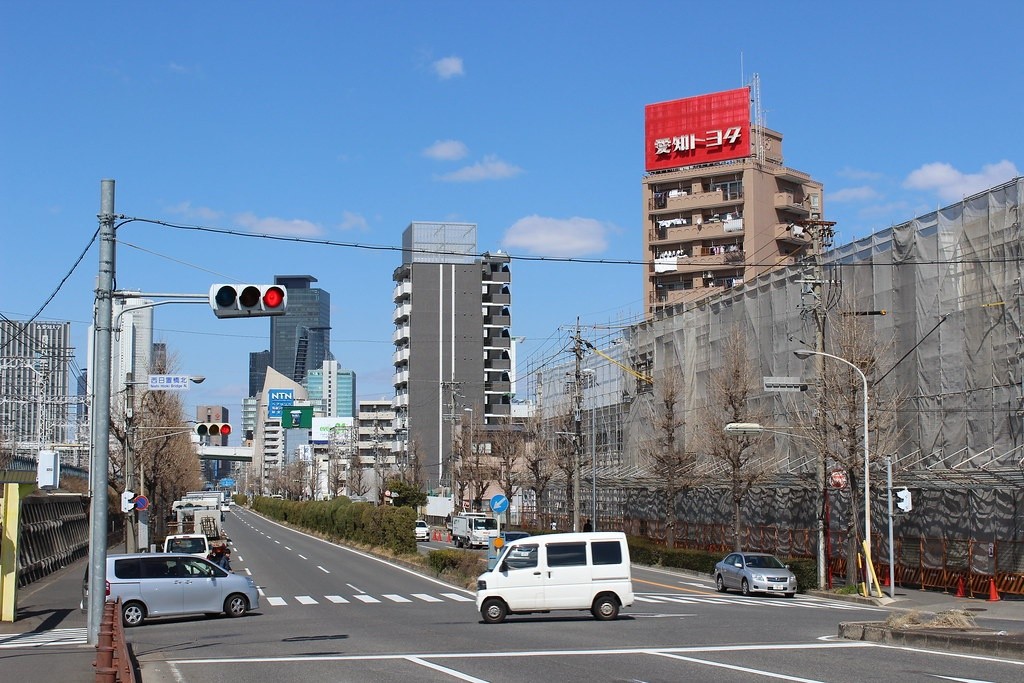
[896,490,912,512]
[121,492,137,513]
[208,284,287,319]
[194,423,232,436]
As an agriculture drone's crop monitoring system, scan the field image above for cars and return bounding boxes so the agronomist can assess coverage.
[413,521,431,542]
[500,532,536,562]
[220,499,236,512]
[714,552,798,597]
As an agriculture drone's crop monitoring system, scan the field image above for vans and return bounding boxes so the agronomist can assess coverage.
[475,532,634,621]
[160,535,210,575]
[79,553,260,626]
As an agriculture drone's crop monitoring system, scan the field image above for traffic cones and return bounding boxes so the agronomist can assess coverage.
[883,567,890,586]
[955,576,966,596]
[432,531,452,543]
[986,579,1000,601]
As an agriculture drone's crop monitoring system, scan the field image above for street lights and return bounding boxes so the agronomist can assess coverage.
[793,349,871,596]
[583,369,596,531]
[138,377,206,554]
[723,422,826,588]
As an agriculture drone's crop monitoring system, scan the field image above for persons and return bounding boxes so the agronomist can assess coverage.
[207,549,234,572]
[583,519,592,532]
[550,520,557,530]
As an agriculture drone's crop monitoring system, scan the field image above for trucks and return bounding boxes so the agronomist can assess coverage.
[446,512,498,549]
